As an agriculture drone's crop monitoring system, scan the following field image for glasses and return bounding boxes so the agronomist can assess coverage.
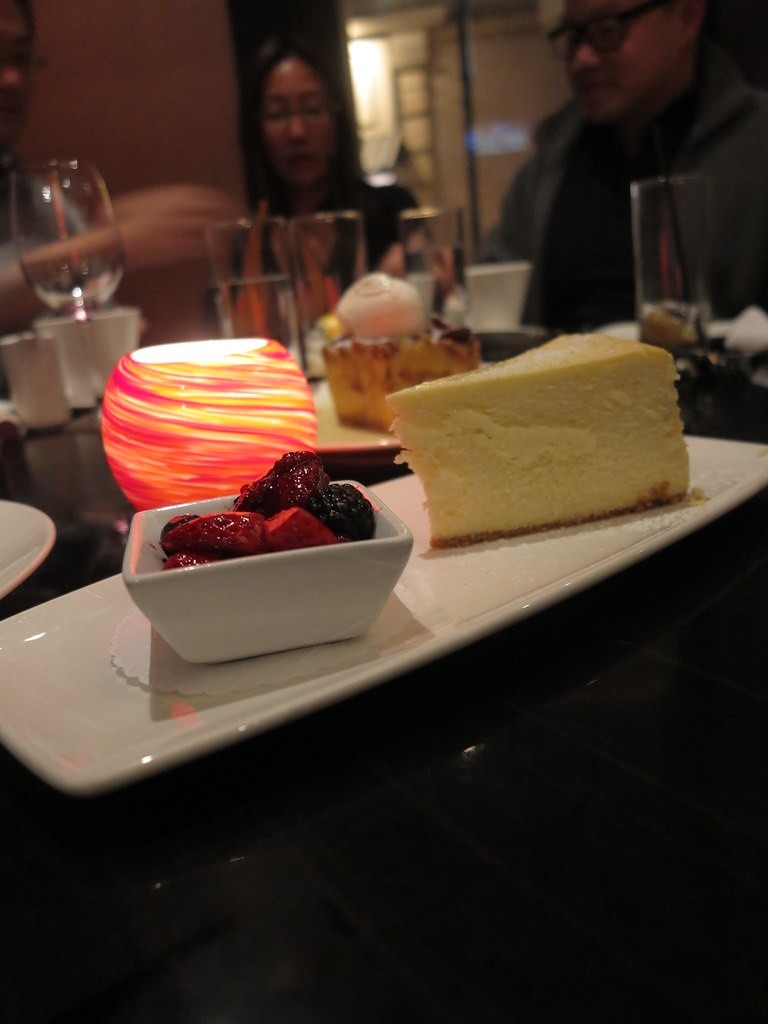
[545,0,663,61]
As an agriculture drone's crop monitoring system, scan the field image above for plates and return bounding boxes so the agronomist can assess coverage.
[306,378,402,469]
[0,499,57,601]
[0,438,768,795]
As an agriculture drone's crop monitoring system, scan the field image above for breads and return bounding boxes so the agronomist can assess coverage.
[387,332,689,546]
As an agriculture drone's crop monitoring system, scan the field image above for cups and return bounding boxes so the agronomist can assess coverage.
[398,204,470,329]
[630,169,719,390]
[202,209,368,380]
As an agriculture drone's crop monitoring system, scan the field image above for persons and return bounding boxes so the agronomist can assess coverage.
[0,0,251,336]
[206,20,462,354]
[372,0,768,446]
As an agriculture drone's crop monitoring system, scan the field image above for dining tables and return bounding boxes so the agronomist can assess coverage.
[0,324,768,1024]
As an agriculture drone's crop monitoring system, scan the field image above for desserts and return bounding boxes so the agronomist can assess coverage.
[322,334,476,431]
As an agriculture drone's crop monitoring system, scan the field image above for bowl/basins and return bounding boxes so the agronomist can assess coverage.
[409,262,534,335]
[31,309,139,400]
[122,479,413,665]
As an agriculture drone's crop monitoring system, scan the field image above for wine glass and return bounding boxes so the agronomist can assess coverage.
[12,159,125,432]
